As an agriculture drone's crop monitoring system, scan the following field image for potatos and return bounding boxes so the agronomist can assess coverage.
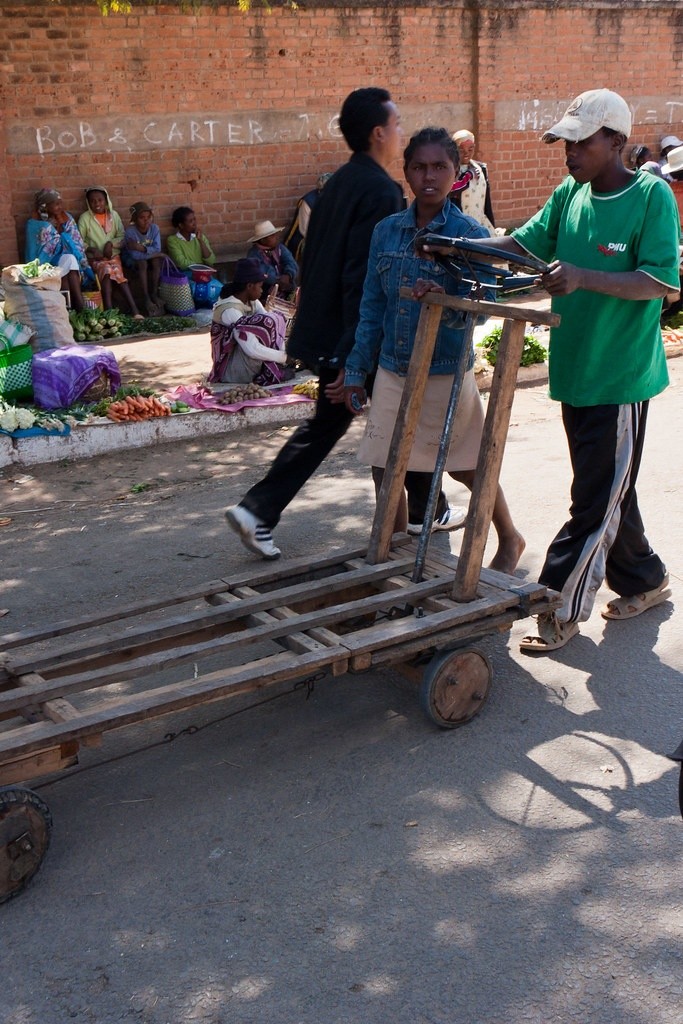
[218,383,272,405]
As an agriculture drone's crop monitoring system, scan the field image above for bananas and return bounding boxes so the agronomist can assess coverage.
[293,379,319,400]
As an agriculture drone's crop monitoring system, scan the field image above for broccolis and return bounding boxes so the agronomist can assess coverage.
[0,407,36,434]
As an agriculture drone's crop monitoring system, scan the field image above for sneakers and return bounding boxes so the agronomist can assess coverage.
[409,509,469,536]
[225,505,280,560]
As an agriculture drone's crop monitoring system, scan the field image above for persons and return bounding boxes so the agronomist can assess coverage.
[23,186,95,309]
[342,127,527,576]
[125,202,168,310]
[168,206,216,267]
[627,135,683,305]
[420,91,680,653]
[78,184,144,320]
[451,130,497,235]
[224,86,481,560]
[246,220,299,299]
[210,256,294,383]
[286,171,335,260]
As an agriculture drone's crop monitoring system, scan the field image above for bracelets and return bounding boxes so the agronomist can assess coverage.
[460,236,471,243]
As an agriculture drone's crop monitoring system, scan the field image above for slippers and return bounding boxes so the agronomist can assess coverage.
[602,577,672,620]
[519,618,580,651]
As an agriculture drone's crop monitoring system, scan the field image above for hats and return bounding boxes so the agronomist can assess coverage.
[128,203,153,222]
[541,85,631,146]
[246,220,286,243]
[635,143,644,163]
[660,147,683,174]
[188,264,218,283]
[660,135,683,156]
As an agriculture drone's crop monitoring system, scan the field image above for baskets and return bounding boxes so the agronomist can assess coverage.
[158,256,195,316]
[78,274,103,312]
[263,283,299,340]
[0,334,35,400]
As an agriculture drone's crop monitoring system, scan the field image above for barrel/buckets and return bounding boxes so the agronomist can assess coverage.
[0,334,35,407]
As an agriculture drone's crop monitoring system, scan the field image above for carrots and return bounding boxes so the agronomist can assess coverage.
[106,397,172,423]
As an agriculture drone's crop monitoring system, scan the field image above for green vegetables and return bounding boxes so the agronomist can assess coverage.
[23,258,53,277]
[70,308,197,340]
[477,329,546,367]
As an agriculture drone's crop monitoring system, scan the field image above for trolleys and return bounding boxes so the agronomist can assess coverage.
[0,233,561,907]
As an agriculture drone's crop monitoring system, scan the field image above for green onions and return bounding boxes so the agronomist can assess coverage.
[29,403,94,432]
